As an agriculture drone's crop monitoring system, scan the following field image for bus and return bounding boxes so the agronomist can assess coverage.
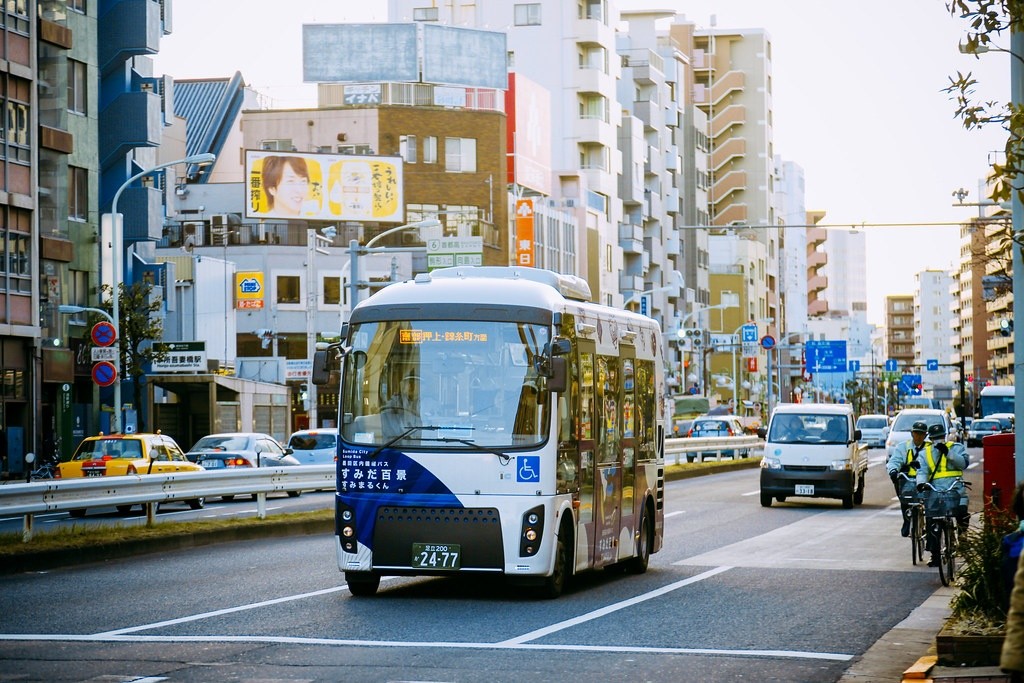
[312,266,663,598]
[981,385,1016,420]
[671,396,717,438]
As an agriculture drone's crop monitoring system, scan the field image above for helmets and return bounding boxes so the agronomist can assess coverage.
[928,425,946,438]
[911,422,927,432]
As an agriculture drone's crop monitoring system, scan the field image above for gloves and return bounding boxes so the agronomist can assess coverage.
[917,484,924,493]
[890,472,897,483]
[910,461,920,469]
[935,443,948,456]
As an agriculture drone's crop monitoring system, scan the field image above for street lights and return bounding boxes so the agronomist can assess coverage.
[871,333,900,415]
[732,318,774,414]
[340,219,441,329]
[681,303,726,394]
[778,330,814,403]
[59,153,216,434]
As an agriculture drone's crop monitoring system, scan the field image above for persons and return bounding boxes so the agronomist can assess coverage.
[261,156,310,217]
[886,422,932,537]
[1000,482,1024,683]
[383,375,437,417]
[914,425,969,567]
[778,417,810,441]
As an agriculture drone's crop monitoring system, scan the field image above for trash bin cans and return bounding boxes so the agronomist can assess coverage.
[984,435,1015,531]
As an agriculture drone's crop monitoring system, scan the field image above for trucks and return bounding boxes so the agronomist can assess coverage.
[757,403,868,509]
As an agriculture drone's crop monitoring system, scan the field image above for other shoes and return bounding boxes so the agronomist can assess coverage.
[958,529,967,542]
[928,561,939,567]
[902,523,910,536]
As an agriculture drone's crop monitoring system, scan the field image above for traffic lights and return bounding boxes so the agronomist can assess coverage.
[1001,318,1011,337]
[966,376,973,382]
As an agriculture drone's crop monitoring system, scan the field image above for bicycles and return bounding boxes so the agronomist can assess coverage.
[896,472,972,587]
[30,460,54,479]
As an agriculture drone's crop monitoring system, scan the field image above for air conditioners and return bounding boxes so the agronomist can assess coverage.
[163,224,180,242]
[183,221,205,245]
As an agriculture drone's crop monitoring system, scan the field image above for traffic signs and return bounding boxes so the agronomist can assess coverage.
[806,340,847,373]
[897,375,922,396]
[741,325,757,342]
[882,370,902,382]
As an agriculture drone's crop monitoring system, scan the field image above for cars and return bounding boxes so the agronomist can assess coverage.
[855,415,892,448]
[983,413,1015,433]
[54,429,207,517]
[951,417,974,442]
[885,409,961,463]
[185,433,302,500]
[686,416,750,462]
[286,427,337,491]
[967,419,1002,448]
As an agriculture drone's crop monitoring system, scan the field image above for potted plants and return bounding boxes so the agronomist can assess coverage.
[936,495,1020,667]
